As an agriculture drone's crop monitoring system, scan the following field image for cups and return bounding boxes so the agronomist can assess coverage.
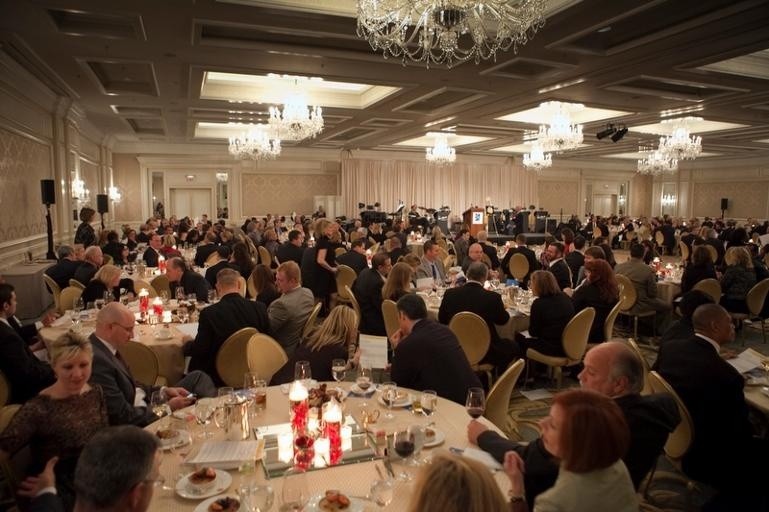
[159,329,172,337]
[254,381,267,415]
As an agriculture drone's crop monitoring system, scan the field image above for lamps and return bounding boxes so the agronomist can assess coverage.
[107,185,122,204]
[216,171,228,183]
[351,0,553,71]
[637,117,704,177]
[424,132,457,166]
[95,192,109,232]
[266,72,329,142]
[39,177,59,260]
[226,123,283,161]
[522,101,587,173]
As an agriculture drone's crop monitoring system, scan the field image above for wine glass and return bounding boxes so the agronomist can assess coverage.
[73,296,85,313]
[218,387,234,405]
[193,398,214,439]
[392,431,411,483]
[247,478,277,511]
[174,286,186,300]
[419,389,438,426]
[85,301,96,317]
[207,288,219,304]
[282,466,310,512]
[294,358,313,391]
[188,293,197,305]
[355,364,375,408]
[236,475,252,512]
[381,381,399,417]
[150,390,169,430]
[242,371,260,401]
[330,358,348,393]
[464,386,485,419]
[177,307,188,330]
[367,471,395,511]
[159,288,171,304]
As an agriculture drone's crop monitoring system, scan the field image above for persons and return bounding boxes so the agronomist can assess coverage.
[501,388,641,512]
[1,201,766,407]
[2,328,110,511]
[467,339,651,512]
[88,302,196,426]
[410,452,506,511]
[654,302,768,512]
[19,424,165,511]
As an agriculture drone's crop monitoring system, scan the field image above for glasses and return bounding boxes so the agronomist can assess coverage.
[112,322,133,331]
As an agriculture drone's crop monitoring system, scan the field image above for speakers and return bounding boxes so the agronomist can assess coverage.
[97,194,108,213]
[721,198,728,210]
[41,179,55,204]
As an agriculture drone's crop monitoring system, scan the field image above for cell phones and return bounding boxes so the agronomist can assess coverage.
[186,391,196,400]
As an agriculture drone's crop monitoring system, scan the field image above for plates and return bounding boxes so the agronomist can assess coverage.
[173,468,232,500]
[375,392,417,408]
[154,335,172,340]
[350,384,376,395]
[194,493,248,512]
[301,488,364,512]
[421,427,447,448]
[163,427,190,449]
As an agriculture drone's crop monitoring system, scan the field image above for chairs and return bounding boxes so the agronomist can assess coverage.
[215,327,260,389]
[40,244,273,315]
[246,332,289,387]
[406,212,423,232]
[643,369,698,500]
[1,403,20,434]
[593,225,769,329]
[118,341,168,387]
[388,204,404,221]
[296,232,380,345]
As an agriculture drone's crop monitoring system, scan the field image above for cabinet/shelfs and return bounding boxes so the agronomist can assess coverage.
[1,255,58,321]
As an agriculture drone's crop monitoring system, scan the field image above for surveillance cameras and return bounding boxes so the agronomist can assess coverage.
[597,127,617,139]
[611,129,628,142]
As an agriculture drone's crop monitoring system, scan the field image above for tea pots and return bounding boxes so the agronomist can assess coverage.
[214,396,255,442]
